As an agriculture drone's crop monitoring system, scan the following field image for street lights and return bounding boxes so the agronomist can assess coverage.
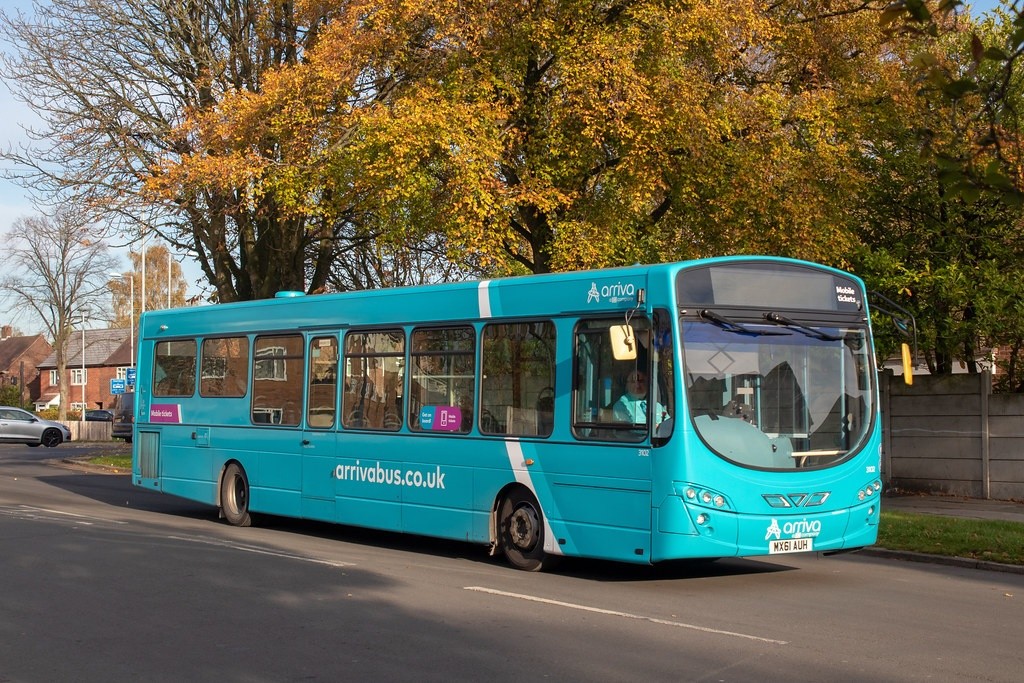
[107,271,134,369]
[166,252,200,358]
[77,305,91,444]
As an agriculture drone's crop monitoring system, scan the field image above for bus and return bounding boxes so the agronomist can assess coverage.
[129,252,916,572]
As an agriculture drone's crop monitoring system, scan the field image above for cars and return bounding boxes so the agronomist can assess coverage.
[80,409,115,422]
[0,405,72,448]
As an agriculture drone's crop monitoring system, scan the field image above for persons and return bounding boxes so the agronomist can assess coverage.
[613,364,671,441]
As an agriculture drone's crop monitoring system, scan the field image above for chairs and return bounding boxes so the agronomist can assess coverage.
[596,408,614,437]
[345,386,554,438]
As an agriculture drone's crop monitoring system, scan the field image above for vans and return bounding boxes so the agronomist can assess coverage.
[111,392,134,445]
